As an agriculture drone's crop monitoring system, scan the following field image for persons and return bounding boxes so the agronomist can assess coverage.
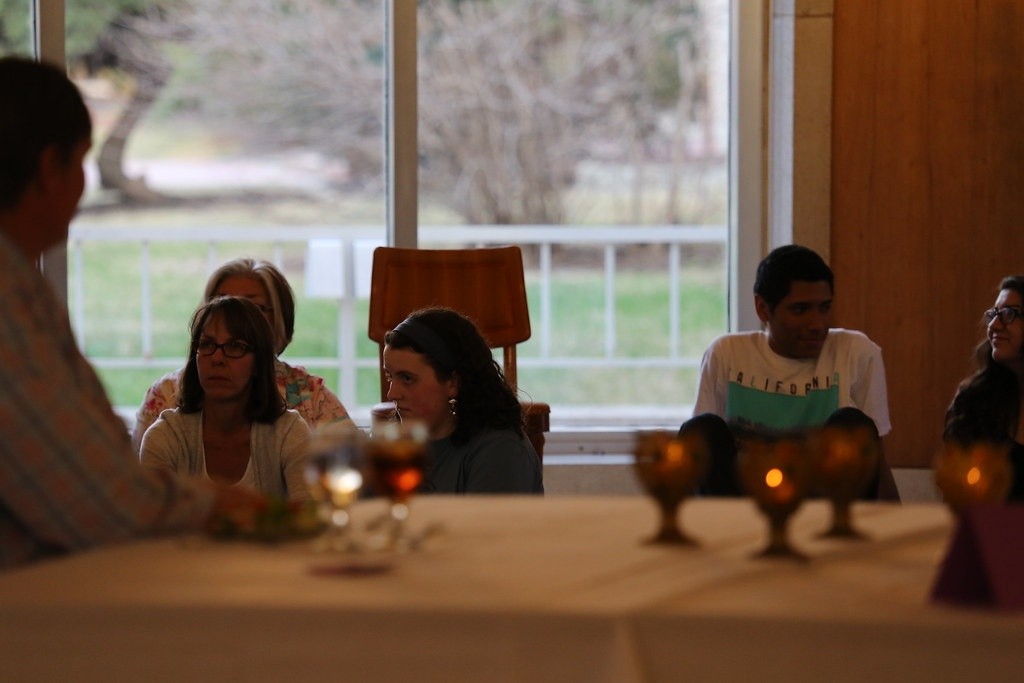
[677,244,901,502]
[131,257,359,449]
[0,55,277,572]
[942,276,1024,471]
[138,297,322,505]
[357,307,545,498]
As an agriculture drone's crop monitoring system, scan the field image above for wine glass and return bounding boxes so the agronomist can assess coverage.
[811,430,877,539]
[304,425,366,556]
[374,424,426,553]
[633,432,707,546]
[733,438,813,565]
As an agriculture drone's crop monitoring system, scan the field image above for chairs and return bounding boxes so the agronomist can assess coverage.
[368,245,552,465]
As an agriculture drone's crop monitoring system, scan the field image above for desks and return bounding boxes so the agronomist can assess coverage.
[0,492,1024,683]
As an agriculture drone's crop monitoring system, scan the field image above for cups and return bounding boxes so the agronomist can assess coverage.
[937,444,1006,517]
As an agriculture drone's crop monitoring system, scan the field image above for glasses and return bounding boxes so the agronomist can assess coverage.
[984,307,1024,325]
[192,339,255,358]
